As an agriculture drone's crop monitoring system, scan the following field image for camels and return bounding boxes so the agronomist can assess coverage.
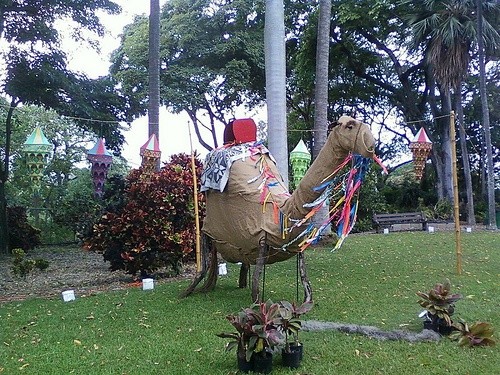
[177,115,375,303]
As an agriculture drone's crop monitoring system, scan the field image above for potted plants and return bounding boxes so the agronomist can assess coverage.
[416,278,463,331]
[216,295,314,374]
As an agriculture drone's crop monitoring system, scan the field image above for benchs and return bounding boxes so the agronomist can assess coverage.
[372,210,427,234]
